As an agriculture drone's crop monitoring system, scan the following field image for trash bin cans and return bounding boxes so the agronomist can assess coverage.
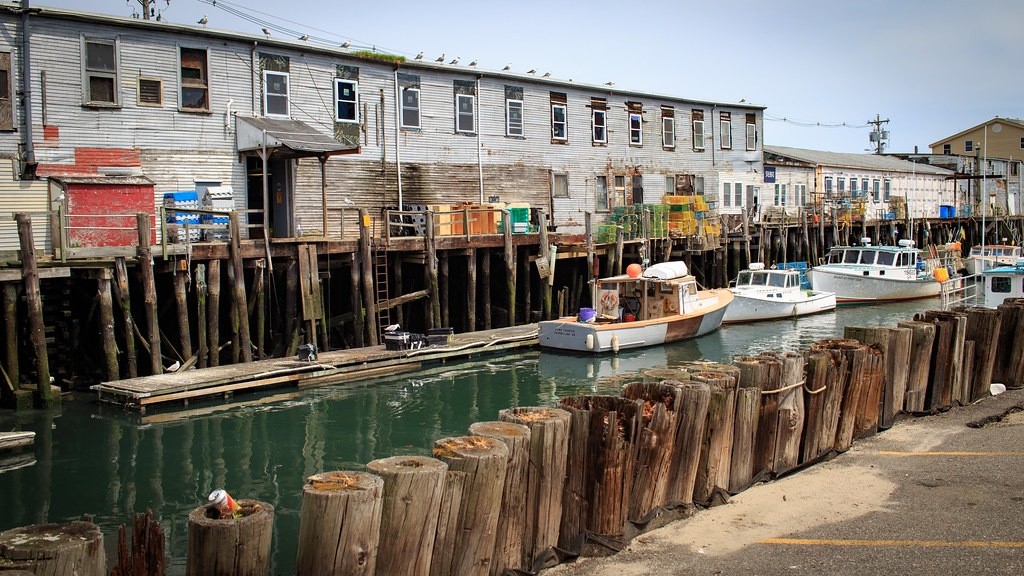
[940,205,956,218]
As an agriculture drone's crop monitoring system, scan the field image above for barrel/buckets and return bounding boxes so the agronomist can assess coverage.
[625,313,635,322]
[949,205,958,218]
[939,205,948,219]
[580,307,595,321]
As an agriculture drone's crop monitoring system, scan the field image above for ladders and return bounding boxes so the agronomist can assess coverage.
[371,238,391,345]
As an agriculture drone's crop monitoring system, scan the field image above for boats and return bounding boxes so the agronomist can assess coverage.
[807,235,963,304]
[938,261,1024,310]
[721,262,837,322]
[537,260,734,354]
[964,245,1024,276]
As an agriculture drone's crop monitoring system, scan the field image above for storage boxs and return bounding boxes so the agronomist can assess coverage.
[162,187,236,243]
[384,326,454,350]
[501,208,530,235]
[595,195,719,243]
